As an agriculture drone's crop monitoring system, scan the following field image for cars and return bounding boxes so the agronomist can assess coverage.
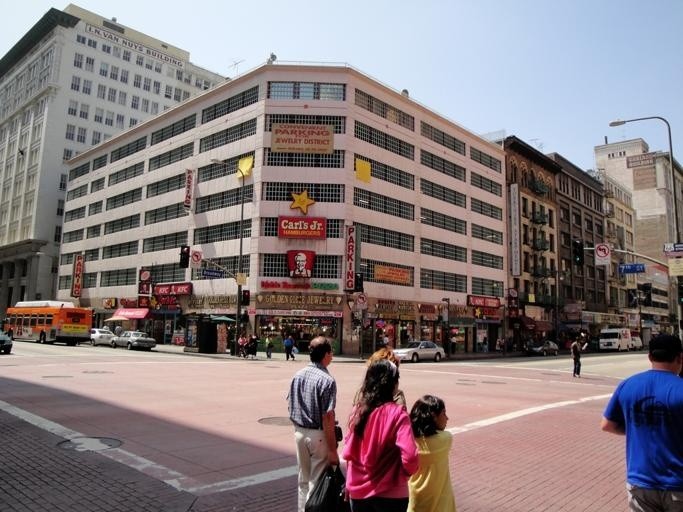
[565,339,589,354]
[110,331,156,350]
[522,340,558,356]
[390,341,447,362]
[629,336,643,351]
[76,328,117,346]
[0,328,12,355]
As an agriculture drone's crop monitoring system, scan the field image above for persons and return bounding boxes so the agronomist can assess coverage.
[345,359,423,512]
[290,335,340,512]
[288,335,293,341]
[247,334,256,359]
[571,337,588,377]
[602,334,682,512]
[265,333,273,358]
[238,333,245,348]
[283,336,294,360]
[407,395,456,512]
[353,346,407,412]
[290,253,312,276]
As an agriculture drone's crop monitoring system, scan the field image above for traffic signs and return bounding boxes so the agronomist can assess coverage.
[619,262,645,274]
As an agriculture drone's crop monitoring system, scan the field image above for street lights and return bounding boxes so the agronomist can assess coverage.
[608,117,681,246]
[208,158,244,356]
[34,251,55,299]
[555,271,565,347]
[441,296,451,357]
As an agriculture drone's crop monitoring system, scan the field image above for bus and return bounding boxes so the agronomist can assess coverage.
[1,301,92,346]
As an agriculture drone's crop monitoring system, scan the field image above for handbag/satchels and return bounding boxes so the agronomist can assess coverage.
[335,424,343,442]
[305,464,352,512]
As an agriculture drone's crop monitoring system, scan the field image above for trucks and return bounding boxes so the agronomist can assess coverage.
[599,327,631,351]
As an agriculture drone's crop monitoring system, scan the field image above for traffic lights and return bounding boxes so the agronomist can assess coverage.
[571,239,584,266]
[242,290,249,306]
[355,273,364,292]
[178,245,190,269]
[677,281,683,306]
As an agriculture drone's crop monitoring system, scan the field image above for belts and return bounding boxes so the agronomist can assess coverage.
[293,421,324,430]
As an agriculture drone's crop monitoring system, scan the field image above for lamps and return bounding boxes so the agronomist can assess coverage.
[318,293,327,304]
[266,291,271,298]
[335,296,342,304]
[347,294,354,310]
[257,294,263,304]
[297,292,302,305]
